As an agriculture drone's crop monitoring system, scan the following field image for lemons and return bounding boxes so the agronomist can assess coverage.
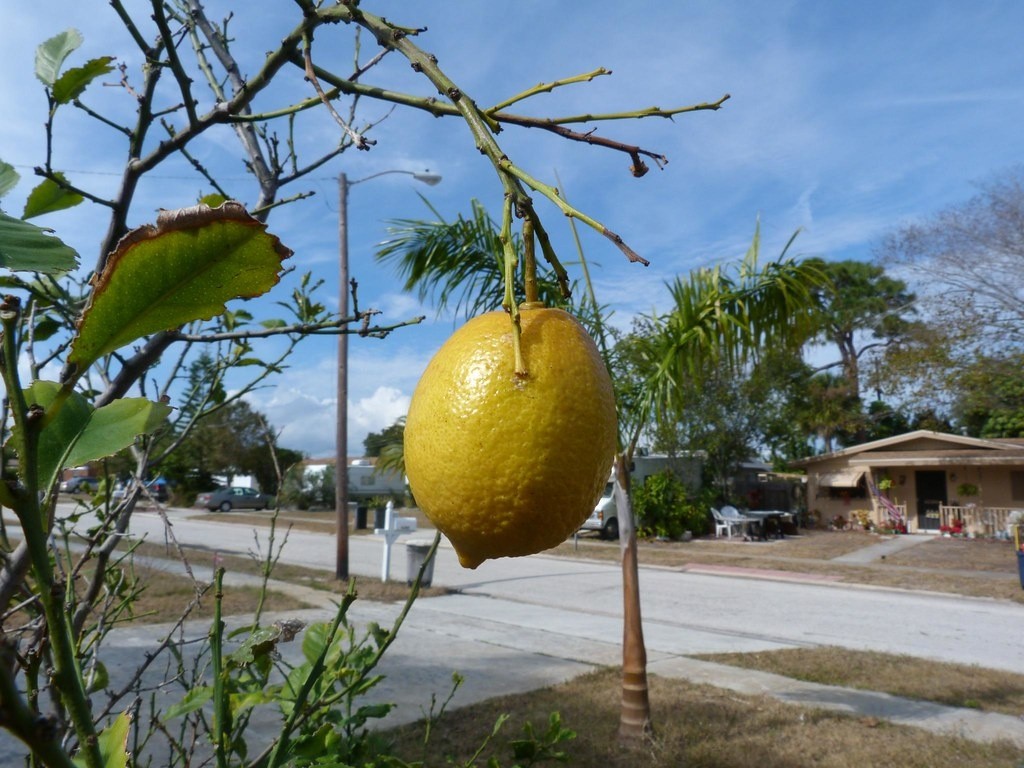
[401,301,616,569]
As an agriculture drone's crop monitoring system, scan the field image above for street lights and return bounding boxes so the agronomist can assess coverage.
[336,167,441,580]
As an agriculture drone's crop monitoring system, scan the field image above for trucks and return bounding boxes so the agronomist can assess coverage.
[300,460,406,509]
[575,447,703,541]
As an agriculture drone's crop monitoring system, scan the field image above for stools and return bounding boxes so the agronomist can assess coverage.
[743,519,768,542]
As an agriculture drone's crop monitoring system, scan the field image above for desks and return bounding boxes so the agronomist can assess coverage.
[744,510,785,542]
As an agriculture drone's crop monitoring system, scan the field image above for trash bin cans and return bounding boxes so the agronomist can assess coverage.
[354,505,369,530]
[373,507,386,529]
[1016,550,1024,589]
[405,540,434,589]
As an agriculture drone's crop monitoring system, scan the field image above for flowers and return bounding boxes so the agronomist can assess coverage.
[879,521,908,535]
[878,480,896,489]
[939,518,963,539]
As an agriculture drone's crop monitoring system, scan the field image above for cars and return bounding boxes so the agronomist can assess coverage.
[113,479,169,502]
[58,476,100,494]
[195,487,277,512]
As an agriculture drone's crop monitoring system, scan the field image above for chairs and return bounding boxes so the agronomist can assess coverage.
[710,507,739,538]
[721,506,740,535]
